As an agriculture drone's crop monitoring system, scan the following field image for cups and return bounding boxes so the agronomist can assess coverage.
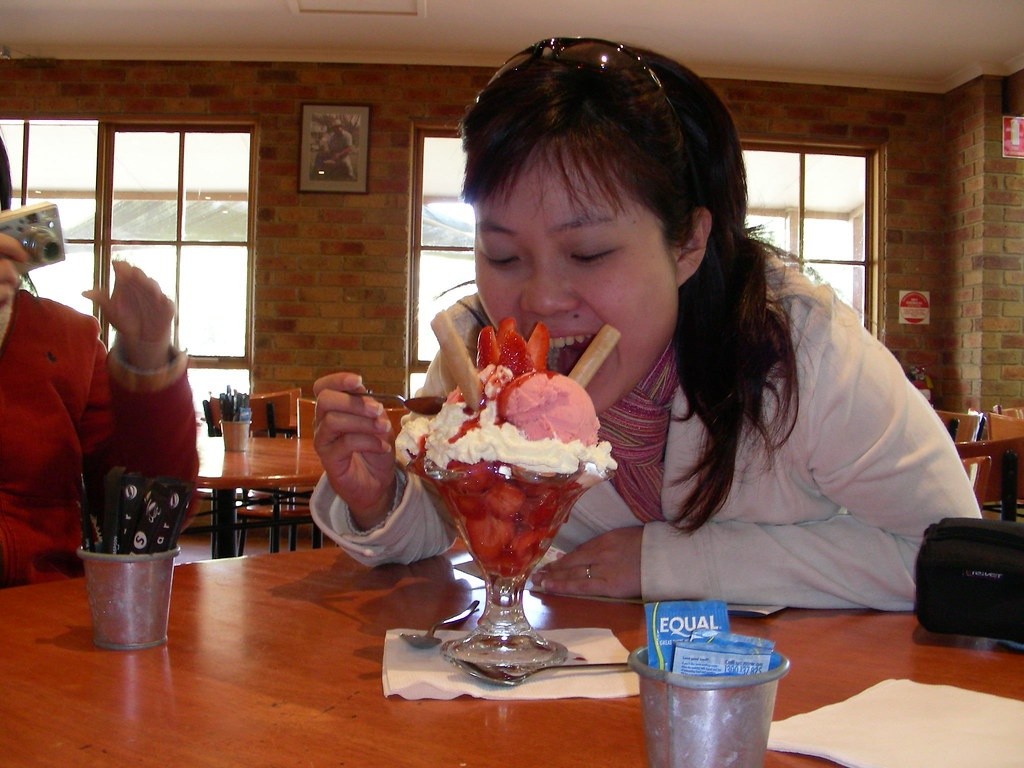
[218,420,252,451]
[76,541,181,650]
[628,646,791,768]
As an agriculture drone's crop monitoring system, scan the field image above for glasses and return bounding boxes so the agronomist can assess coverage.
[472,37,689,164]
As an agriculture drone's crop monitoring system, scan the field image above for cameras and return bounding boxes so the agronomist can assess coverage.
[0,201,65,273]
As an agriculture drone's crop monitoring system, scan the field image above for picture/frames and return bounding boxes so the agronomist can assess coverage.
[299,102,372,194]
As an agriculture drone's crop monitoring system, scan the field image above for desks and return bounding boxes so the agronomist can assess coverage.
[183,436,324,558]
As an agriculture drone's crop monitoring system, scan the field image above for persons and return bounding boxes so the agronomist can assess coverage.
[0,133,200,595]
[309,37,983,614]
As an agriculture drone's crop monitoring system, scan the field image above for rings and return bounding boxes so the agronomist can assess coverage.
[586,565,591,578]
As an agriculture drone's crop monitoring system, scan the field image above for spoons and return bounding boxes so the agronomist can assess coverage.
[342,391,446,414]
[453,658,632,687]
[399,598,478,649]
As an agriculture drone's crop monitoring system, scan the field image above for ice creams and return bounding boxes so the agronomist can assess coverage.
[392,311,622,478]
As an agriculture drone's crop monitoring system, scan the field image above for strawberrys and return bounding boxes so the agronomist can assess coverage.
[476,316,552,376]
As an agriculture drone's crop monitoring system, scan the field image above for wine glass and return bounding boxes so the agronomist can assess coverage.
[406,450,614,675]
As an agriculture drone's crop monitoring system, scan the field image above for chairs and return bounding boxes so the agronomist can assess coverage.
[201,387,411,558]
[935,405,1024,524]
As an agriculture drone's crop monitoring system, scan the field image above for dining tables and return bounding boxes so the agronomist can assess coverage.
[0,546,1024,768]
